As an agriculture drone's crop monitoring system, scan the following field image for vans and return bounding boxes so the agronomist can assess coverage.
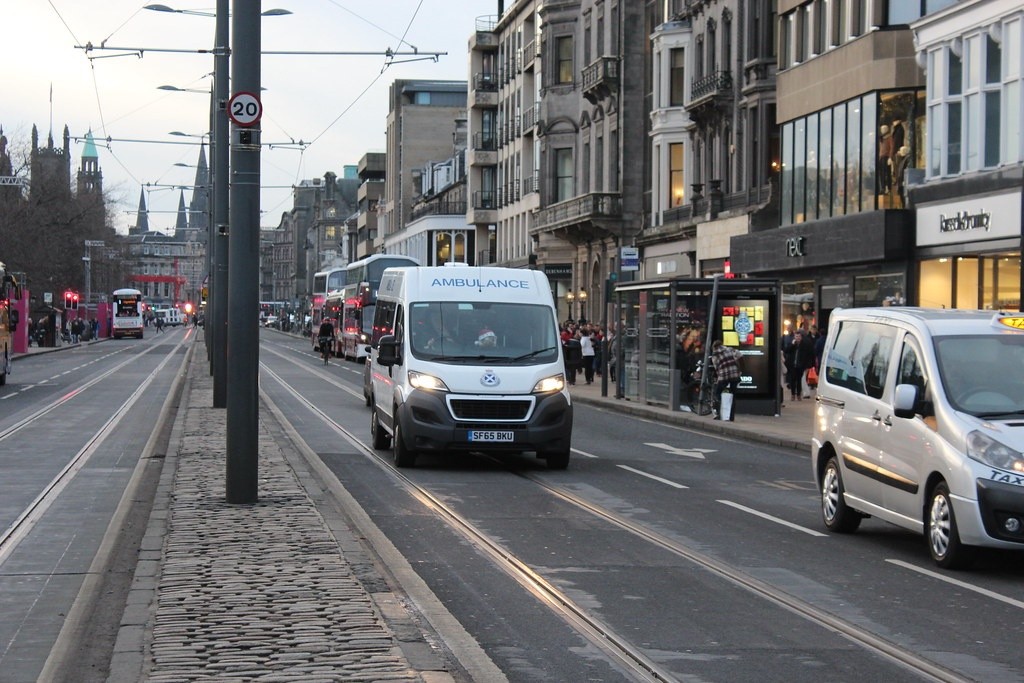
[811,305,1024,572]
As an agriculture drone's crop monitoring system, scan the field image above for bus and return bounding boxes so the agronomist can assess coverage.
[199,301,209,315]
[311,267,347,358]
[259,301,313,320]
[113,288,144,339]
[342,253,423,363]
[0,263,22,387]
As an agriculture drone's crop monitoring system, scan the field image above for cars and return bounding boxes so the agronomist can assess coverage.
[180,314,188,323]
[360,346,371,406]
[265,316,277,327]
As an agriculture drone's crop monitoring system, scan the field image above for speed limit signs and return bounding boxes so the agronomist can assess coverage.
[227,91,262,126]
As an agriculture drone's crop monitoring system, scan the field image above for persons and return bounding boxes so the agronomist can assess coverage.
[193,314,198,328]
[711,340,741,422]
[156,316,164,334]
[317,318,335,358]
[71,316,85,344]
[558,319,625,387]
[783,322,826,401]
[88,316,100,340]
[416,309,466,349]
[107,312,111,337]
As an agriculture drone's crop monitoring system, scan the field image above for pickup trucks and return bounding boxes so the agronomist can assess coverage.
[276,316,294,331]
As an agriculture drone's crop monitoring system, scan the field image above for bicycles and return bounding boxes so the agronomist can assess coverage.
[317,335,335,365]
[685,355,722,420]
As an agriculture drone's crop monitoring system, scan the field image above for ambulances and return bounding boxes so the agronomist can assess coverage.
[369,264,575,471]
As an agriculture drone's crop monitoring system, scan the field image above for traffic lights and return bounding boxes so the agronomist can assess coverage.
[72,294,78,310]
[65,292,72,309]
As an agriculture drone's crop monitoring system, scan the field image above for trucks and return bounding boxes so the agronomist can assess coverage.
[154,309,180,327]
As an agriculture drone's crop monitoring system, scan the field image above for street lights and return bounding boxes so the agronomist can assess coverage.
[142,4,293,411]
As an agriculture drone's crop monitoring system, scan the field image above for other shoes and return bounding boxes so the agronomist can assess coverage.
[797,395,802,401]
[730,415,734,420]
[713,414,718,419]
[680,405,691,412]
[791,395,795,401]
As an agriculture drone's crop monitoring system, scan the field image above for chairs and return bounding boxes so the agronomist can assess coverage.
[943,357,979,393]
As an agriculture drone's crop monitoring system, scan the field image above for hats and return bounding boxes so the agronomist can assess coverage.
[325,316,330,319]
[479,329,496,341]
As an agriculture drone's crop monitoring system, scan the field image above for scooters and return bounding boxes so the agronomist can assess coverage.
[303,328,312,337]
